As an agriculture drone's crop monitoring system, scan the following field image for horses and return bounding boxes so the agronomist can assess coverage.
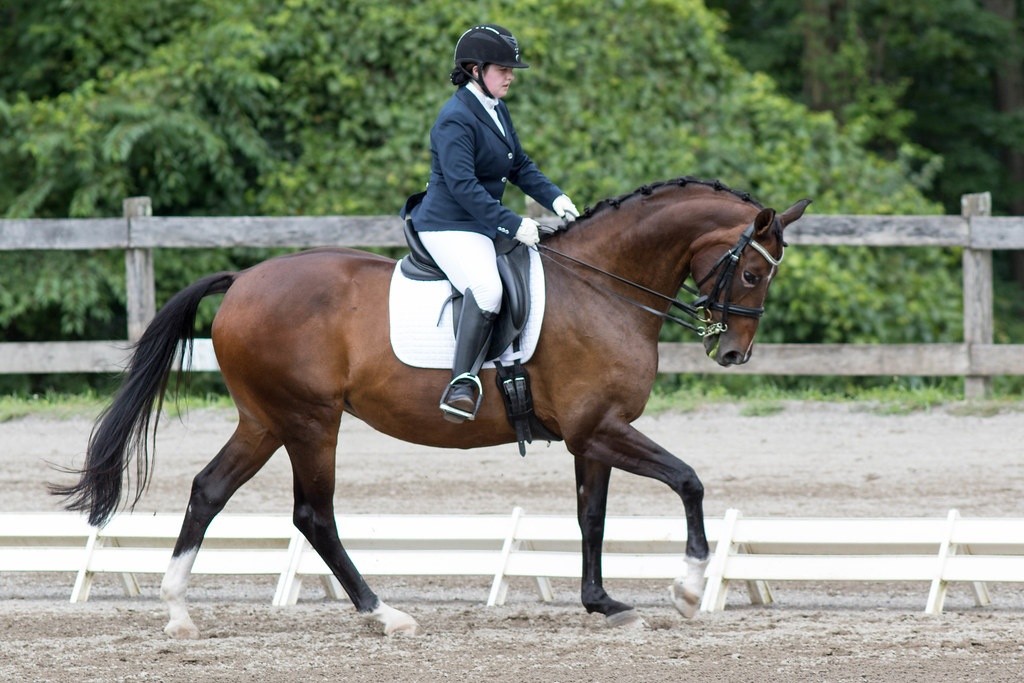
[42,177,812,636]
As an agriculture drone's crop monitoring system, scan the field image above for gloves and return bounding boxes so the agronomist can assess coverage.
[514,218,540,250]
[552,194,580,223]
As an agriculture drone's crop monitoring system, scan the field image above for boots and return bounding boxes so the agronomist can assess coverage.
[443,287,499,423]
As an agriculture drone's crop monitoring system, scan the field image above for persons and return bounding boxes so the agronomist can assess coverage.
[410,26,580,423]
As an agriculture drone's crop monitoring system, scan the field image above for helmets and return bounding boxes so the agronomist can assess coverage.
[454,25,529,68]
